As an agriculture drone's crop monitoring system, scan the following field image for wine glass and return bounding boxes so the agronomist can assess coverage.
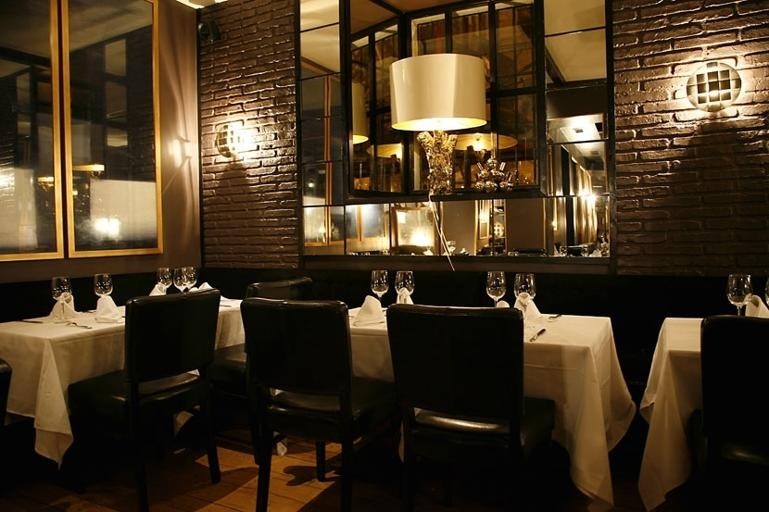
[446,240,457,256]
[51,273,72,323]
[394,270,414,304]
[486,270,507,307]
[157,266,198,294]
[94,273,114,320]
[514,272,536,335]
[371,269,389,301]
[727,274,752,315]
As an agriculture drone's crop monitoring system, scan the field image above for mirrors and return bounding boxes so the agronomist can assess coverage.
[0,1,67,262]
[59,0,166,257]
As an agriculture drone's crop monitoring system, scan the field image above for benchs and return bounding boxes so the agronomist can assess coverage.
[203,267,768,481]
[1,266,203,459]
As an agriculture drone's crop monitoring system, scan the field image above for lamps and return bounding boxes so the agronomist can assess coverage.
[215,124,244,157]
[447,128,518,151]
[389,53,488,194]
[352,84,369,145]
[687,61,740,114]
[366,138,403,158]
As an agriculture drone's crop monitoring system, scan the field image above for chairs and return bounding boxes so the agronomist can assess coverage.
[700,314,768,510]
[352,243,609,258]
[383,302,557,509]
[68,289,222,510]
[239,297,392,511]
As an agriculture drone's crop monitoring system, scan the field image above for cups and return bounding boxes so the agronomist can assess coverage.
[763,277,769,307]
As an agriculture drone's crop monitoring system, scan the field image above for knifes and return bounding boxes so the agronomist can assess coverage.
[531,328,546,341]
